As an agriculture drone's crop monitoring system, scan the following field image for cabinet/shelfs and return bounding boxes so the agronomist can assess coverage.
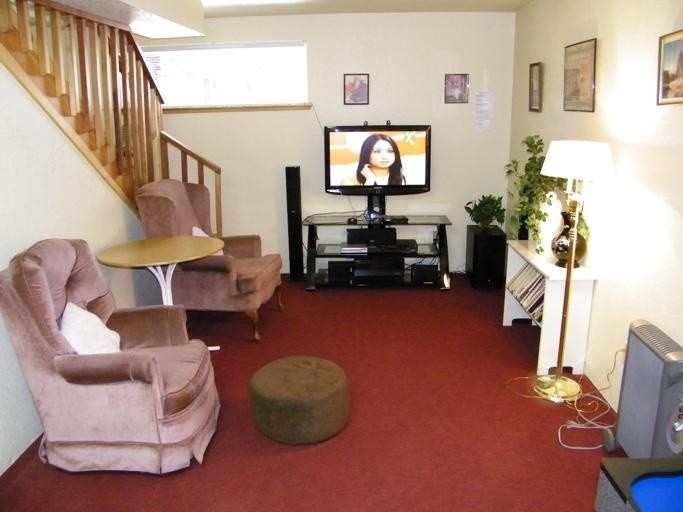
[501,239,597,377]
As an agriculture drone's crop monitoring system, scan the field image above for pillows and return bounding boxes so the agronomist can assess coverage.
[192,226,224,256]
[60,302,122,354]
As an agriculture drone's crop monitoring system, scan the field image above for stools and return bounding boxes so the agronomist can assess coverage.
[248,354,346,446]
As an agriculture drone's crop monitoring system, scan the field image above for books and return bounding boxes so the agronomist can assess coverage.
[506,263,545,328]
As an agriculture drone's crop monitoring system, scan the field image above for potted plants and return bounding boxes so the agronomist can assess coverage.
[464,135,587,267]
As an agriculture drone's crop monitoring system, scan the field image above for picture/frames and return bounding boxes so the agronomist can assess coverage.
[343,74,369,105]
[444,74,469,103]
[657,29,682,105]
[564,38,597,112]
[528,62,543,112]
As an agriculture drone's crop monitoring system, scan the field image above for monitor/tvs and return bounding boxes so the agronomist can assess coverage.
[325,125,431,224]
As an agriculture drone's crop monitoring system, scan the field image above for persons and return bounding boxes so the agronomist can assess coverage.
[338,134,406,186]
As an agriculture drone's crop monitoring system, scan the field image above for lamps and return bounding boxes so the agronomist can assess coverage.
[533,140,617,403]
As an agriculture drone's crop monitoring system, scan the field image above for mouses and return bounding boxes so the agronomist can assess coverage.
[347,217,356,223]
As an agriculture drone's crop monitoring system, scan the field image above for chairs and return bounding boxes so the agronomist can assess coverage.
[134,178,291,340]
[0,239,221,476]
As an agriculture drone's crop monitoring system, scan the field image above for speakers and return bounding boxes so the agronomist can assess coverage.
[285,166,303,279]
[329,261,353,285]
[412,265,437,286]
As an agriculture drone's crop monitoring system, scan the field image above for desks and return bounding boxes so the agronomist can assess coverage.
[301,213,451,291]
[95,236,224,353]
